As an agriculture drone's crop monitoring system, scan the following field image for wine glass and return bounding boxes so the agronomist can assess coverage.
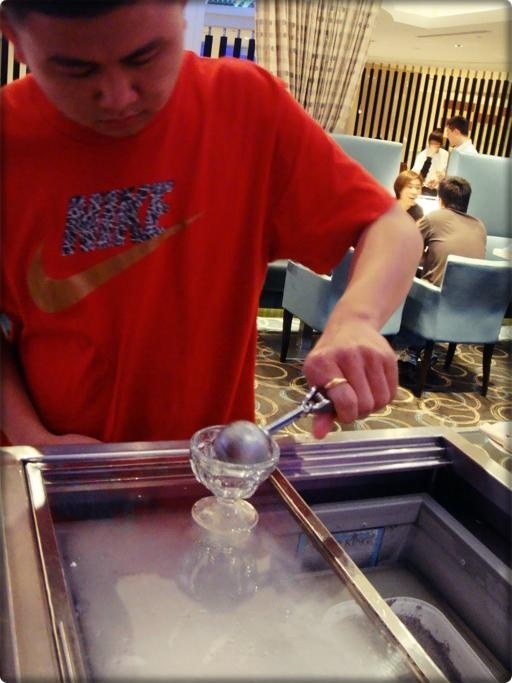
[189,424,281,535]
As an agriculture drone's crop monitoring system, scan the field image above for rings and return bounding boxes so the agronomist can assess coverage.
[322,375,349,390]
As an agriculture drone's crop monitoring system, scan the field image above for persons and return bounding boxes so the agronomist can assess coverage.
[401,174,488,370]
[0,0,426,450]
[391,169,424,225]
[421,169,445,195]
[444,115,482,158]
[407,130,451,191]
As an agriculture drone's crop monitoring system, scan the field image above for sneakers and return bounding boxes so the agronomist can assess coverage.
[420,350,439,366]
[397,348,418,366]
